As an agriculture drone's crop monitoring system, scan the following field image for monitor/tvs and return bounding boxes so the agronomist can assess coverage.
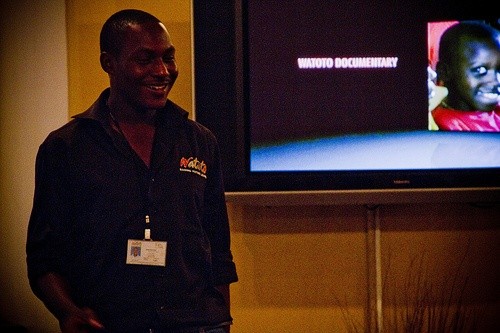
[195,0,499,204]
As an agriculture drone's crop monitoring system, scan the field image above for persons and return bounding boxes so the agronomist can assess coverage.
[26,8,239,333]
[428,21,500,133]
[131,247,139,257]
